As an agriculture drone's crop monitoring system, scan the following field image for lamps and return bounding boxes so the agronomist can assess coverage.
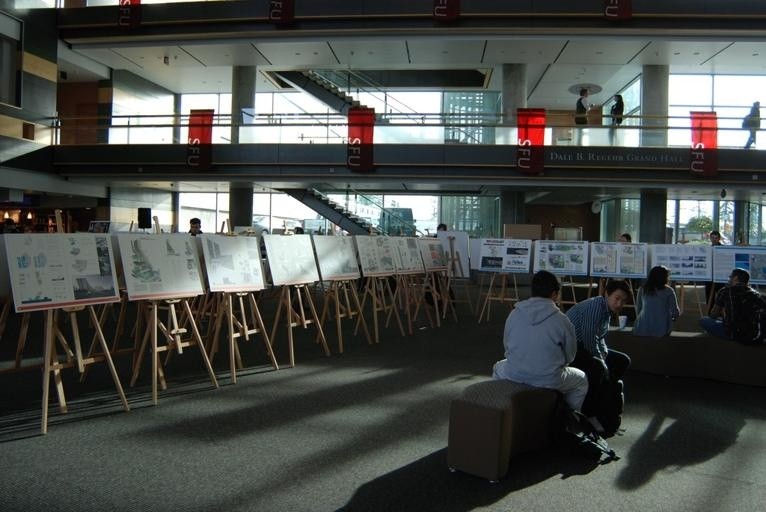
[3,211,9,219]
[27,211,32,220]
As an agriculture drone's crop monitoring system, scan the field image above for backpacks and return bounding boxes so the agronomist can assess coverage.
[725,284,766,345]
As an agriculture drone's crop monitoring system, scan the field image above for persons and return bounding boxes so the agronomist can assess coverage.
[493,270,589,422]
[294,227,303,233]
[620,234,639,303]
[700,268,766,346]
[430,224,456,312]
[633,266,680,337]
[566,280,631,382]
[0,218,36,233]
[742,102,760,149]
[188,218,203,236]
[608,95,624,146]
[706,231,726,316]
[575,89,595,145]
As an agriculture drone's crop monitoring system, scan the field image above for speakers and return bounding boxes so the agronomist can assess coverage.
[138,208,151,228]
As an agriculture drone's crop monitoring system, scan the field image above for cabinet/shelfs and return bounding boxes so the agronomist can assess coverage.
[35,209,66,233]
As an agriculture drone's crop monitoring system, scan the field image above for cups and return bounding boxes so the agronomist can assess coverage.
[619,315,627,329]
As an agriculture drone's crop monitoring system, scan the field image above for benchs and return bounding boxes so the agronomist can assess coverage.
[445,376,624,485]
[604,325,766,390]
